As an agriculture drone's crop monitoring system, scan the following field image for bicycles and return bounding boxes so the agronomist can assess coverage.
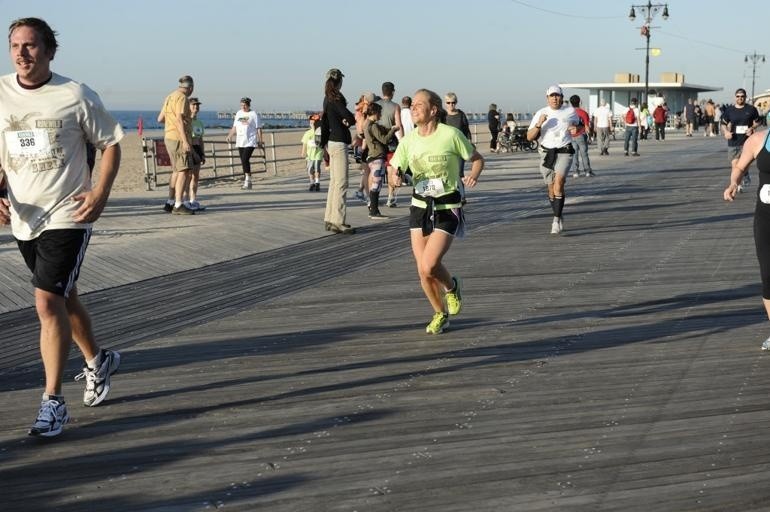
[492,126,540,154]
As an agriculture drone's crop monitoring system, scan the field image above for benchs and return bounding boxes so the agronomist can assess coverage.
[495,125,538,152]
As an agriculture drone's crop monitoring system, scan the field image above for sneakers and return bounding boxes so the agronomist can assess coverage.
[624,152,641,156]
[599,150,610,156]
[444,275,463,314]
[640,139,665,143]
[240,181,252,190]
[573,172,595,178]
[762,335,770,350]
[162,201,208,215]
[354,191,397,219]
[309,182,320,191]
[686,132,716,137]
[81,351,120,405]
[550,222,564,234]
[426,312,450,335]
[28,393,73,437]
[737,176,750,192]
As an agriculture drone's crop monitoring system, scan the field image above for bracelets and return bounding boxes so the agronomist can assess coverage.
[535,123,541,129]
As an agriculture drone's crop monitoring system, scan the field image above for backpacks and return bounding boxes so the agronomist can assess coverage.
[625,106,637,124]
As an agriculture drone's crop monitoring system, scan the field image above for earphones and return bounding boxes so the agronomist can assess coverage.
[431,110,434,115]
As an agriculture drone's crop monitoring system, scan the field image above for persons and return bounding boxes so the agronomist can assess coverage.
[593,98,614,156]
[622,86,770,193]
[226,97,262,190]
[184,97,205,211]
[0,16,125,438]
[526,86,584,237]
[296,68,473,235]
[488,100,517,152]
[569,95,595,178]
[156,75,195,215]
[386,87,485,335]
[723,128,770,352]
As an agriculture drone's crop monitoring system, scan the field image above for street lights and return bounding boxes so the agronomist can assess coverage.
[628,2,669,103]
[741,50,766,102]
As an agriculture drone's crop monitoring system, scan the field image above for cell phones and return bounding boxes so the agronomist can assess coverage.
[397,167,408,185]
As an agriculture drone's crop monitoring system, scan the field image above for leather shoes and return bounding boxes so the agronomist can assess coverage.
[325,221,355,234]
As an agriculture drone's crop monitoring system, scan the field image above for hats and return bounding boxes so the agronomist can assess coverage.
[325,68,344,80]
[631,98,638,102]
[735,88,746,94]
[547,85,563,96]
[189,98,202,106]
